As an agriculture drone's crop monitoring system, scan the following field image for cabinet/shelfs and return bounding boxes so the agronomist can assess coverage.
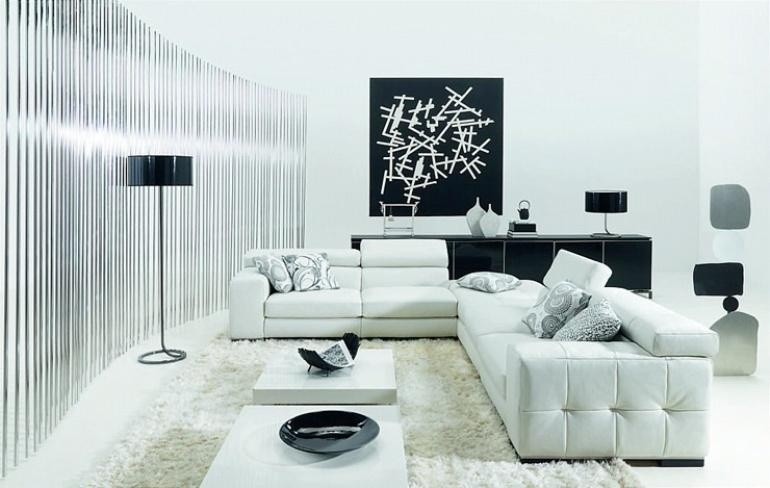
[352,233,652,300]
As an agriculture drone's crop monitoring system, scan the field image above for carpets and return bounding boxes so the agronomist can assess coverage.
[81,329,649,486]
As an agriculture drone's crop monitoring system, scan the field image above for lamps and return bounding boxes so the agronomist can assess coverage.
[125,155,193,364]
[585,188,629,238]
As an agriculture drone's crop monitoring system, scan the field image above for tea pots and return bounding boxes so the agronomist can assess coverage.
[516,199,531,219]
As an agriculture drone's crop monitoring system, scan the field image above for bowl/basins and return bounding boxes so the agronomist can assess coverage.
[278,410,381,456]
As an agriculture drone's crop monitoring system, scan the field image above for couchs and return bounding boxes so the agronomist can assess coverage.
[228,237,719,466]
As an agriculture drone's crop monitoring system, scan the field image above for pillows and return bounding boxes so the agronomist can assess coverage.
[457,271,520,293]
[521,278,590,338]
[551,297,622,342]
[282,250,341,291]
[253,250,293,293]
